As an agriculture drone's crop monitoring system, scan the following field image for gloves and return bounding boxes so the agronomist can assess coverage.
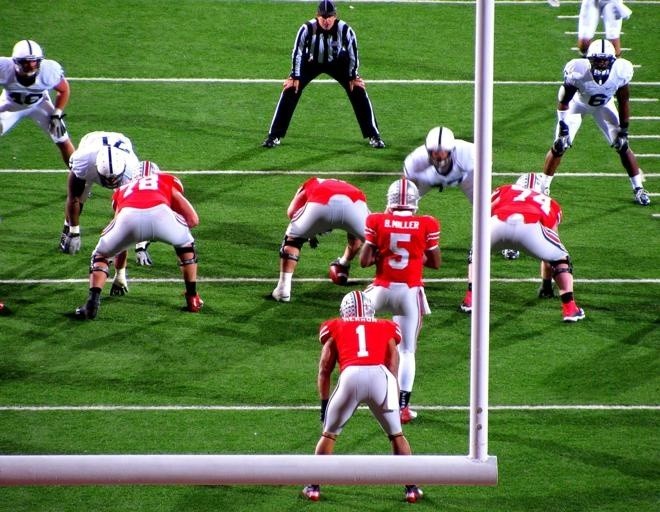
[321,400,329,421]
[548,0,560,8]
[538,282,558,299]
[110,268,130,296]
[135,241,151,266]
[64,231,81,256]
[550,132,571,154]
[611,131,630,154]
[48,116,66,138]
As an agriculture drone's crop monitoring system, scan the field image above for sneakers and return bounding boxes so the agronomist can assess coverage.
[634,188,651,205]
[58,226,68,251]
[184,290,204,313]
[74,302,99,319]
[369,135,386,147]
[263,134,280,147]
[459,247,585,322]
[399,404,418,425]
[272,282,291,303]
[302,484,321,502]
[406,485,424,503]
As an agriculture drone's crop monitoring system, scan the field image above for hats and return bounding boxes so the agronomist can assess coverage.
[317,1,335,17]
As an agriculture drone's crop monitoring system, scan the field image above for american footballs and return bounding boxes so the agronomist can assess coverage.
[329,264,349,284]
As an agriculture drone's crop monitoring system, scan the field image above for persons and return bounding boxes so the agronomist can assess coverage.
[300,290,425,505]
[271,176,373,303]
[261,0,386,149]
[0,39,76,171]
[459,172,586,323]
[403,126,520,261]
[359,174,443,425]
[540,37,651,206]
[57,130,153,268]
[74,160,205,320]
[546,0,624,58]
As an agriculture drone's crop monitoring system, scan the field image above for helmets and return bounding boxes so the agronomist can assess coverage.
[132,161,159,181]
[426,125,455,167]
[96,146,126,189]
[516,173,544,194]
[388,177,419,214]
[12,39,42,79]
[587,39,616,81]
[340,291,376,318]
